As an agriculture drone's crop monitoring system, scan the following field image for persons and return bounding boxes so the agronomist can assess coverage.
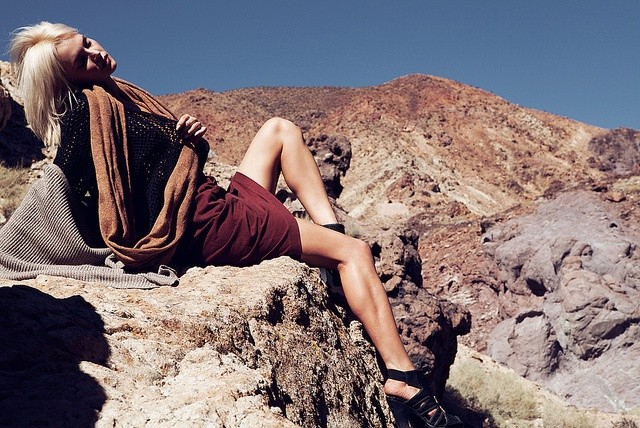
[1,20,468,427]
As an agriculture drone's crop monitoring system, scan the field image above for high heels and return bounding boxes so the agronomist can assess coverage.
[385,367,485,428]
[320,223,349,309]
